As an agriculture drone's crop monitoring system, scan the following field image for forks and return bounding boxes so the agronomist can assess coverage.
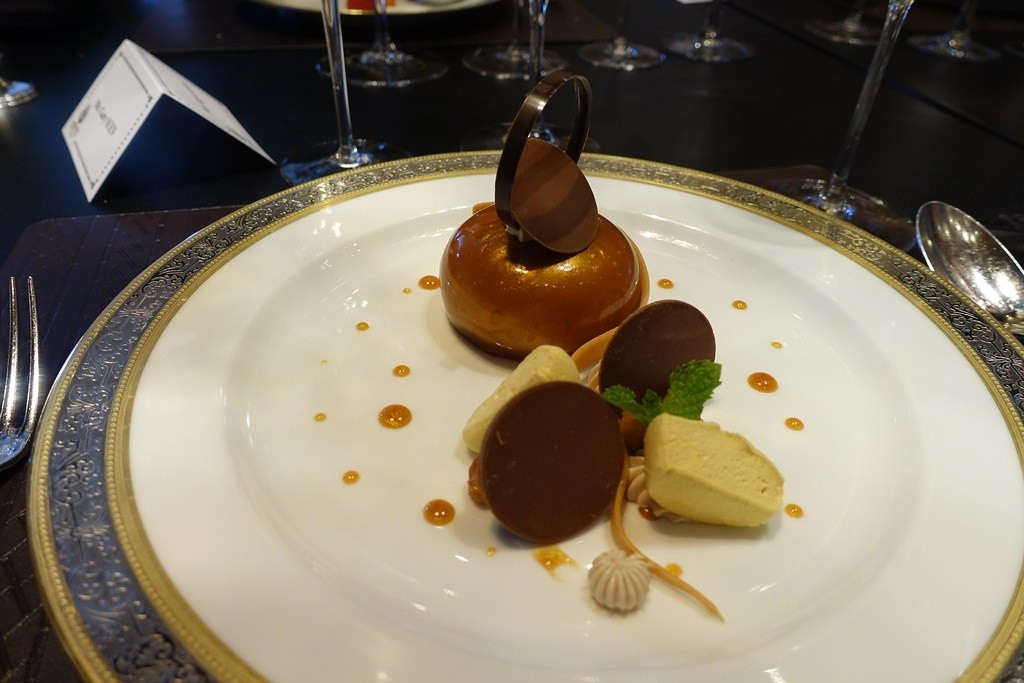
[1,277,43,464]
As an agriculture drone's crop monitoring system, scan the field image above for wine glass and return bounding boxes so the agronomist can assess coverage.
[279,0,1006,255]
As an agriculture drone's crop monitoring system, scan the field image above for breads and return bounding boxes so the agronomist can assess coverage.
[640,413,787,529]
[460,345,582,450]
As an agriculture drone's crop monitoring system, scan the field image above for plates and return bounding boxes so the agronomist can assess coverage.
[25,146,1024,680]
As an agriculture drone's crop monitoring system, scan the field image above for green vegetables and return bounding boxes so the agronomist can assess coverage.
[601,359,722,421]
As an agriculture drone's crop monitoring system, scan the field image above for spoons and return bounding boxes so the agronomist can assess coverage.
[915,199,1023,340]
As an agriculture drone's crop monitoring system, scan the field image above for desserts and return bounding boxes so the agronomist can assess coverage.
[439,69,651,361]
